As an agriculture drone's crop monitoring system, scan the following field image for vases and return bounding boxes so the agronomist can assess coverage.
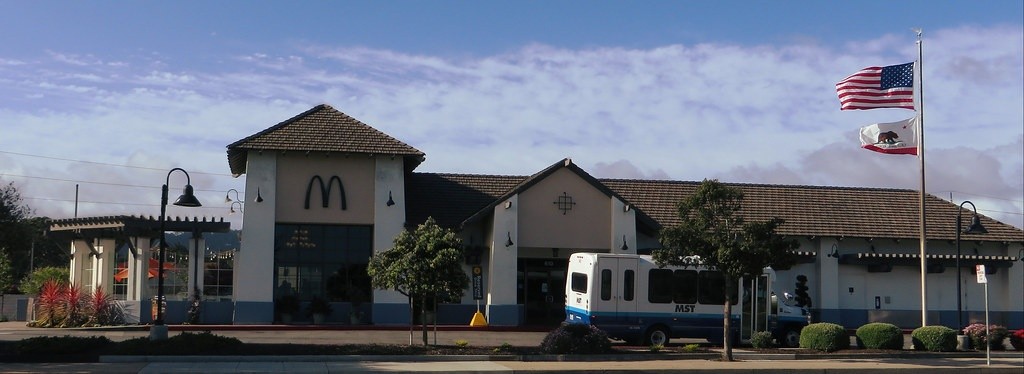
[1010,340,1024,350]
[968,338,1005,350]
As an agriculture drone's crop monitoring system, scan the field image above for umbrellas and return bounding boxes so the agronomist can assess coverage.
[114,268,168,283]
[118,256,175,271]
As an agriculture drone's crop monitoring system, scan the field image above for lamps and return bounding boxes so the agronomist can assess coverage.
[505,233,514,248]
[225,189,244,203]
[828,243,840,259]
[1016,249,1024,262]
[621,236,628,250]
[256,188,264,203]
[849,287,854,295]
[284,229,317,249]
[168,251,189,263]
[386,191,395,206]
[229,201,244,214]
[206,246,236,263]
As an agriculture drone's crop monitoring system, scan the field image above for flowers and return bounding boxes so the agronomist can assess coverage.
[962,324,1006,344]
[1007,326,1024,339]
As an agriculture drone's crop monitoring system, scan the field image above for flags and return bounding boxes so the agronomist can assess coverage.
[835,59,918,113]
[860,117,920,157]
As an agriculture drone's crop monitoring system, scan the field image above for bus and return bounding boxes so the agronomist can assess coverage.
[561,252,813,348]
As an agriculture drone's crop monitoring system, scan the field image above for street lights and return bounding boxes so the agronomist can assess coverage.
[956,200,990,335]
[287,227,316,292]
[151,167,203,325]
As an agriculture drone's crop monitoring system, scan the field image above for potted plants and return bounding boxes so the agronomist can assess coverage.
[275,292,301,325]
[305,294,334,325]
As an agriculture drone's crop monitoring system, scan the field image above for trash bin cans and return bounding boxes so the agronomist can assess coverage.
[151,295,167,321]
